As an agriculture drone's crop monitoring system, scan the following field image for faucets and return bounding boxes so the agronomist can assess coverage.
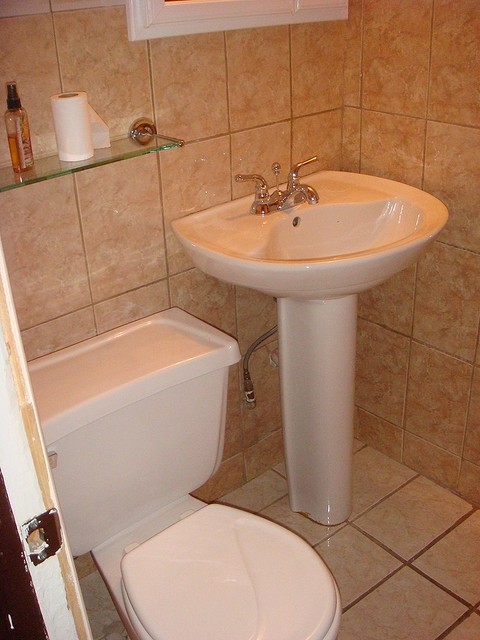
[234,156,320,215]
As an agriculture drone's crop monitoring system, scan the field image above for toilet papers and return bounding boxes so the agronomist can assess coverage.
[48,90,113,163]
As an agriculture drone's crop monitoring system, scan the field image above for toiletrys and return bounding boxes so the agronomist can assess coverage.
[3,79,37,174]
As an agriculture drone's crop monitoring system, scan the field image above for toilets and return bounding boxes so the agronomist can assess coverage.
[25,304,345,640]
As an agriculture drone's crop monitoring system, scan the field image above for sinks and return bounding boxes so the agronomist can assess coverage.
[169,168,451,301]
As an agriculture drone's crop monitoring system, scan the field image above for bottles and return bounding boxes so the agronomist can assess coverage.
[5,78,34,174]
